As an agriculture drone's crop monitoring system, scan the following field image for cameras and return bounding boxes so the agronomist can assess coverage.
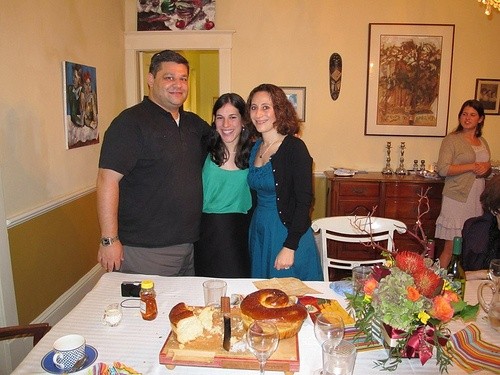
[121,281,142,297]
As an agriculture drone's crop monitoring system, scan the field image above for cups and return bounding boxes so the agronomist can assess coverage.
[321,338,358,375]
[104,303,123,326]
[489,259,500,281]
[53,334,86,371]
[202,279,227,306]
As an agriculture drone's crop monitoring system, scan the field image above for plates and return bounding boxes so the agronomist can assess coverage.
[41,345,98,374]
[334,170,355,176]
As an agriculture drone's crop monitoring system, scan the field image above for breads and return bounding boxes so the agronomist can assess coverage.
[169,302,215,344]
[239,288,308,341]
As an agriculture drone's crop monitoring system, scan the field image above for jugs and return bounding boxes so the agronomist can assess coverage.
[477,278,500,332]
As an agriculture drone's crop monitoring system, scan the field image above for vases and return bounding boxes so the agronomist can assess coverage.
[382,322,419,358]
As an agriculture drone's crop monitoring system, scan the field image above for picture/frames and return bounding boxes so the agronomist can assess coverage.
[475,78,500,115]
[280,87,306,122]
[364,23,456,138]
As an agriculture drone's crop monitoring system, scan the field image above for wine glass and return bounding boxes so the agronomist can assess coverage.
[315,313,344,375]
[245,321,280,374]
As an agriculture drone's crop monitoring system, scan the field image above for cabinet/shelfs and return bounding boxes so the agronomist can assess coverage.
[325,171,444,281]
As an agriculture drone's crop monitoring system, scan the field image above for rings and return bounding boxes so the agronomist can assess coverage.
[285,267,290,270]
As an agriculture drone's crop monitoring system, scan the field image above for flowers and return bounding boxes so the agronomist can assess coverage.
[342,249,482,375]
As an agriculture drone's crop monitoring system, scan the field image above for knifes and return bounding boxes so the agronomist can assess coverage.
[221,296,232,351]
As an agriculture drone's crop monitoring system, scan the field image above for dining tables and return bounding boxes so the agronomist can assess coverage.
[10,272,500,375]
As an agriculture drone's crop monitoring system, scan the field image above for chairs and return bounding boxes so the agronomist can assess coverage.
[0,323,51,375]
[311,215,407,281]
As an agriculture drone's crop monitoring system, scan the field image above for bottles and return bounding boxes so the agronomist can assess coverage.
[448,237,466,302]
[139,280,158,321]
[425,239,436,266]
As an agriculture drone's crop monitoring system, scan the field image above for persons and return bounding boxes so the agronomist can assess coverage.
[246,83,323,281]
[479,88,495,109]
[436,100,492,272]
[461,181,500,272]
[193,92,252,278]
[95,49,210,277]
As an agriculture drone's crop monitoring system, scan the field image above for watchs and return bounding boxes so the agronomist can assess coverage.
[100,235,120,246]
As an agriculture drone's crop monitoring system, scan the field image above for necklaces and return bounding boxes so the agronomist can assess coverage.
[259,141,276,158]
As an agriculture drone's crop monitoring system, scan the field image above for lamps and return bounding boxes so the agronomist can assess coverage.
[477,0,500,16]
